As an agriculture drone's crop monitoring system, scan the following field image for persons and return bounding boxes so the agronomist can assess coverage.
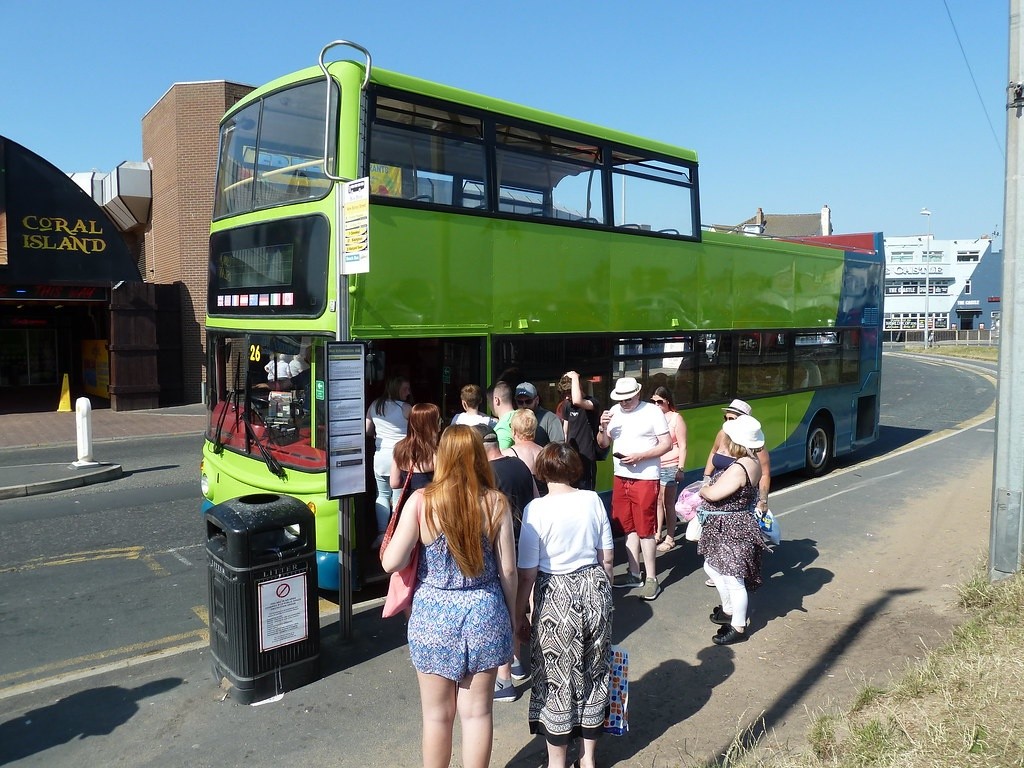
[255,344,323,410]
[517,444,615,768]
[265,353,304,398]
[388,403,441,505]
[651,385,687,553]
[503,410,551,499]
[705,400,753,587]
[383,424,519,768]
[474,423,535,703]
[485,380,519,451]
[450,384,502,430]
[598,377,673,598]
[558,371,597,491]
[515,381,565,447]
[697,416,773,646]
[365,376,414,546]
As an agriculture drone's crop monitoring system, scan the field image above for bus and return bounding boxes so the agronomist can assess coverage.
[201,40,887,592]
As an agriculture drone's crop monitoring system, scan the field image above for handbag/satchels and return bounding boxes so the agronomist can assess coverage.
[380,514,398,561]
[757,508,781,545]
[602,644,629,736]
[381,488,424,618]
[686,516,703,541]
[675,480,710,522]
[709,605,732,623]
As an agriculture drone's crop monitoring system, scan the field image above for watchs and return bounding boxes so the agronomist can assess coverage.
[677,467,685,472]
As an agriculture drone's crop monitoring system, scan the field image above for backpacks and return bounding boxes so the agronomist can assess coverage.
[566,394,611,461]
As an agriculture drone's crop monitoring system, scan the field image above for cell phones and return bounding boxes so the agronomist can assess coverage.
[613,452,625,459]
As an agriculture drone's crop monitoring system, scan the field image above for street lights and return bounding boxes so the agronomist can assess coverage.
[920,206,933,351]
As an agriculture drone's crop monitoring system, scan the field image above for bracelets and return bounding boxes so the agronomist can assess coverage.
[705,474,710,477]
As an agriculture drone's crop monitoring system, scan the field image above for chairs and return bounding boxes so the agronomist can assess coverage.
[795,360,810,388]
[806,360,822,387]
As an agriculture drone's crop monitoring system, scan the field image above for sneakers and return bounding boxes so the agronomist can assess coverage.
[612,568,644,588]
[639,576,661,599]
[712,623,748,644]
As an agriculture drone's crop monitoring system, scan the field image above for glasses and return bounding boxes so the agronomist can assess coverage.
[723,415,734,421]
[515,397,536,405]
[649,399,667,405]
[437,415,442,426]
[617,398,632,402]
[508,423,516,431]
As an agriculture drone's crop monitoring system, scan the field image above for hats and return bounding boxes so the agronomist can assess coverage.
[610,377,642,400]
[723,415,765,448]
[721,399,752,416]
[515,382,537,398]
[472,423,498,442]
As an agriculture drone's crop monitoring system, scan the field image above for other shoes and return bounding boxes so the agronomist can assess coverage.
[510,662,526,680]
[654,531,666,545]
[746,574,764,591]
[492,684,517,702]
[705,578,716,586]
[657,541,675,551]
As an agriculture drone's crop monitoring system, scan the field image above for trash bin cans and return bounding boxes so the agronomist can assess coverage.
[204,493,323,706]
